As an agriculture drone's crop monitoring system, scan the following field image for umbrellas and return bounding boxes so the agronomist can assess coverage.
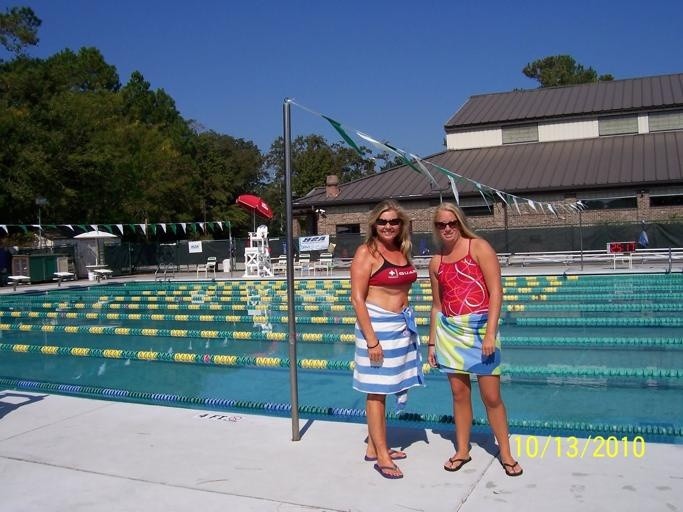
[73,230,118,265]
[236,194,273,233]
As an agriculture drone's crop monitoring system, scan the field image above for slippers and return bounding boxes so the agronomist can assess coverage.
[500,461,523,476]
[444,456,471,472]
[365,450,407,479]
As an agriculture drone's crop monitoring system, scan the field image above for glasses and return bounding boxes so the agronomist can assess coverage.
[376,219,401,225]
[435,220,458,229]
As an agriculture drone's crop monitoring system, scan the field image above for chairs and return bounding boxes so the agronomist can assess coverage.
[271,252,333,276]
[197,256,217,280]
[247,224,269,252]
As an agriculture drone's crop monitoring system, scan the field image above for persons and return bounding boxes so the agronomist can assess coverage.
[426,203,524,476]
[350,200,426,479]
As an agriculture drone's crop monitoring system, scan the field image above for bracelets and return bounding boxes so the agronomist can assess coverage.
[367,339,379,349]
[428,344,435,346]
